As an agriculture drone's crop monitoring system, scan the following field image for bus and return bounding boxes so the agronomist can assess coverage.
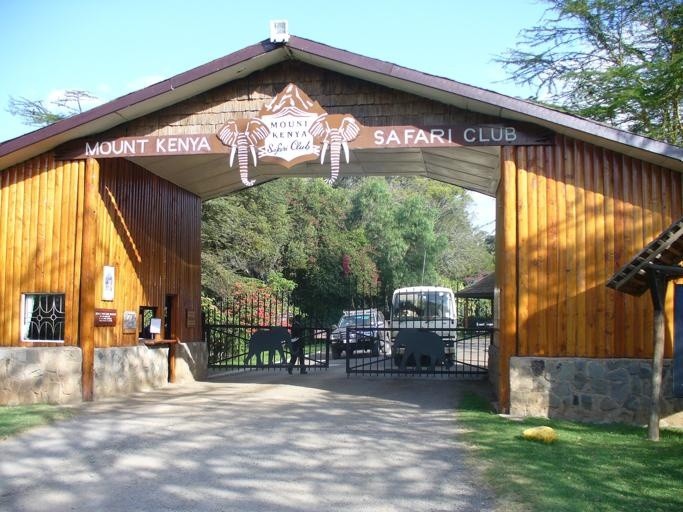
[389,286,457,367]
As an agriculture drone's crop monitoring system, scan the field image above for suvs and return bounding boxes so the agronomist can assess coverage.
[329,309,389,356]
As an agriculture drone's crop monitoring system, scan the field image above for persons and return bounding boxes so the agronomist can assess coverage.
[287,315,308,375]
